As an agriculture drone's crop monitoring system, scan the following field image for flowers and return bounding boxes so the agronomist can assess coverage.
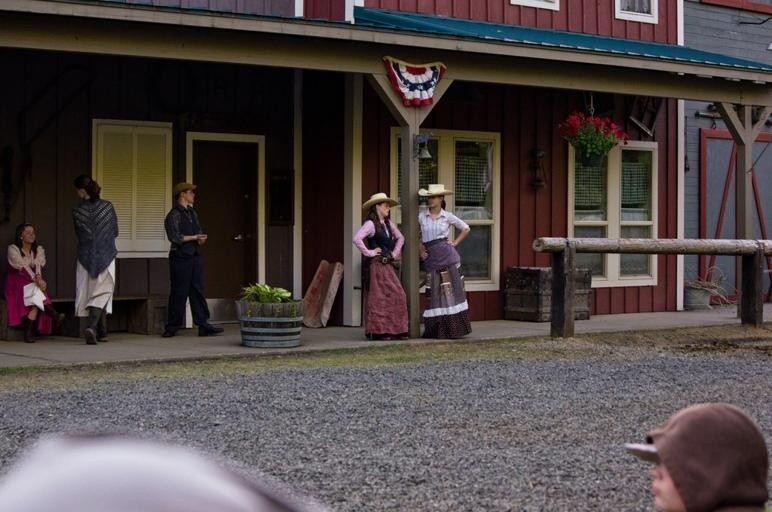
[554,112,629,155]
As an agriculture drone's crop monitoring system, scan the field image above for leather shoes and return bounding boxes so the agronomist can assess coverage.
[199,323,224,336]
[162,330,175,337]
[380,335,391,340]
[398,334,409,340]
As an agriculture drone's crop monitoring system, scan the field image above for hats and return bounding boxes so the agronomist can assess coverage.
[362,192,399,210]
[624,443,660,463]
[174,182,197,195]
[418,184,454,197]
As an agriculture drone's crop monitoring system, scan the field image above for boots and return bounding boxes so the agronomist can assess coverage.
[44,304,65,331]
[84,306,103,345]
[21,313,36,343]
[97,308,109,342]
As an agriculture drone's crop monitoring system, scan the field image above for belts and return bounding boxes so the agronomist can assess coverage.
[377,255,395,264]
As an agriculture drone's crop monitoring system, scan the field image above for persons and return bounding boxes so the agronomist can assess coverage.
[352,191,410,340]
[4,223,66,342]
[163,181,225,338]
[623,400,772,512]
[72,174,119,345]
[417,183,474,340]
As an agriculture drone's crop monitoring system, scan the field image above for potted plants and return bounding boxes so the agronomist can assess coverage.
[234,281,303,349]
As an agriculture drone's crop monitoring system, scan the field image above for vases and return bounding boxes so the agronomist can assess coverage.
[582,151,602,166]
